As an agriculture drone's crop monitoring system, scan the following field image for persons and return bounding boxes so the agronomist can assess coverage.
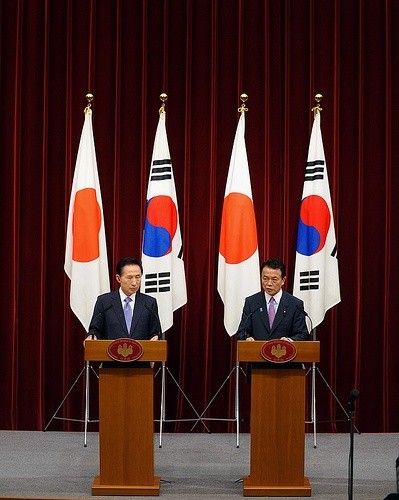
[237,258,313,342]
[83,257,162,346]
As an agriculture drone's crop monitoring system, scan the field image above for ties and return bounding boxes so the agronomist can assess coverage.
[123,296,140,331]
[268,297,276,330]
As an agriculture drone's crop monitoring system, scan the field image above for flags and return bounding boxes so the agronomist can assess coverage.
[62,108,111,333]
[294,110,341,334]
[215,108,262,337]
[142,110,189,333]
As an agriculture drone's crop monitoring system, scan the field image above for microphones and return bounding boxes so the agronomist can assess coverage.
[243,304,262,340]
[349,389,359,403]
[92,304,113,340]
[144,304,161,340]
[296,305,312,341]
